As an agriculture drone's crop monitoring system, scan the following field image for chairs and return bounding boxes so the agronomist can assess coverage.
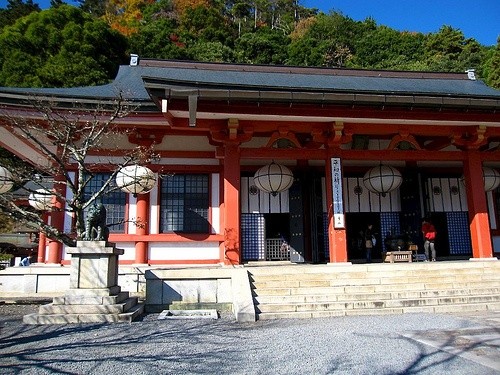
[410,244,419,263]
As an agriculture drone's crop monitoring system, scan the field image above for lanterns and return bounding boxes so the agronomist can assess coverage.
[363,166,402,197]
[254,164,294,196]
[116,165,156,198]
[0,166,13,195]
[460,166,500,192]
[29,188,62,212]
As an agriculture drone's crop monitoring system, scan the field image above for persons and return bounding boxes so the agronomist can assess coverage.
[365,224,374,263]
[384,232,393,251]
[420,217,437,261]
[273,233,285,243]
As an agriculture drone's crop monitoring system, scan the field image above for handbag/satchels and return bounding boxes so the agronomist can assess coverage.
[365,240,372,248]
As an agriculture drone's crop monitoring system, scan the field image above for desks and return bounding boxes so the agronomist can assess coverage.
[382,250,412,263]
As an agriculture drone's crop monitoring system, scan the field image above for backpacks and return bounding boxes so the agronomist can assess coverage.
[424,224,435,240]
